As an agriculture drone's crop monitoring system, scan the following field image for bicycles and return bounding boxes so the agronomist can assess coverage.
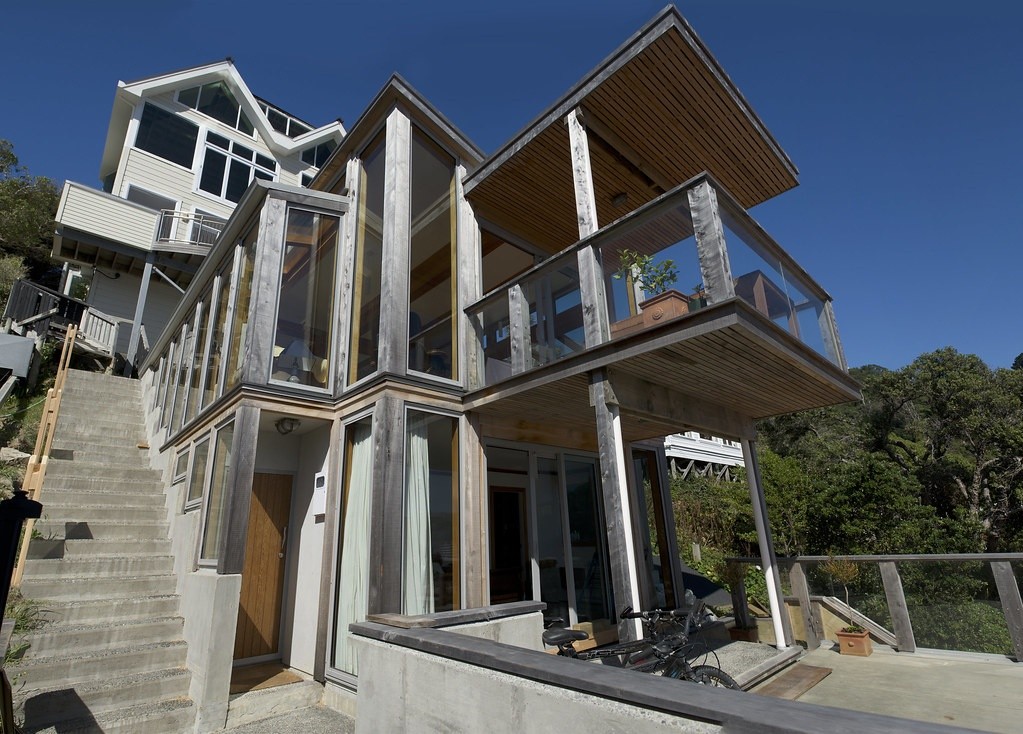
[543,599,743,692]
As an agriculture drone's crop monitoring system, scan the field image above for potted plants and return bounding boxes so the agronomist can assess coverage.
[612,247,693,328]
[686,281,707,312]
[834,625,874,658]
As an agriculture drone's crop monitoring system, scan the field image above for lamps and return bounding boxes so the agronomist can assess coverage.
[279,338,313,384]
[275,418,302,436]
